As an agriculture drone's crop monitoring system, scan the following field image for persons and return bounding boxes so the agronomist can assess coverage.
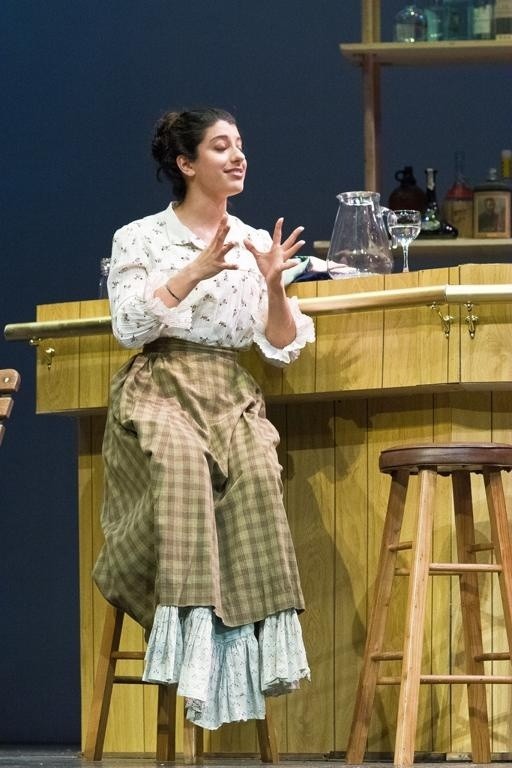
[92,106,316,730]
[478,198,499,232]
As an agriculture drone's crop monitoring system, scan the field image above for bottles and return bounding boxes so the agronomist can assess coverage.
[390,1,495,43]
[98,257,111,300]
[389,150,473,240]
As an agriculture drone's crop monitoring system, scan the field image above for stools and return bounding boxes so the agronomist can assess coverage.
[345,447,512,768]
[84,603,280,766]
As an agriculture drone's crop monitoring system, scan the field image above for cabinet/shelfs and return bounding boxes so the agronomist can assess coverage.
[313,1,512,268]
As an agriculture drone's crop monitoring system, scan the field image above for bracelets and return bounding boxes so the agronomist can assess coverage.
[165,283,182,302]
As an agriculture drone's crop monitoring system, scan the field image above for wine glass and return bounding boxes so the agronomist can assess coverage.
[388,208,422,273]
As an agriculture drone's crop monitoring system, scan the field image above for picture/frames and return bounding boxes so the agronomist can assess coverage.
[473,188,511,239]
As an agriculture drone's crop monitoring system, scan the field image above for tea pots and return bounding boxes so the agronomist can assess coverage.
[326,190,397,279]
